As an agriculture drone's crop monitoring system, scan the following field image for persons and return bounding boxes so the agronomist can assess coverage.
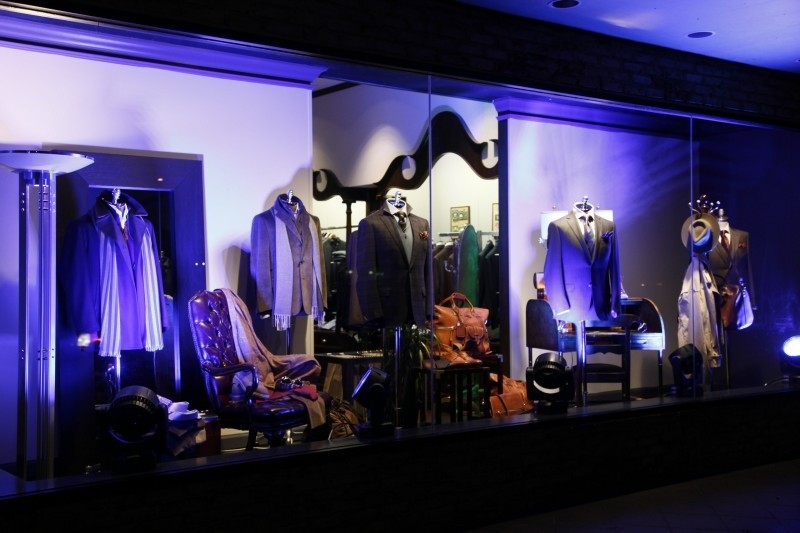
[458,226,479,308]
[545,203,621,324]
[74,189,170,355]
[357,197,435,327]
[251,194,328,331]
[705,222,756,322]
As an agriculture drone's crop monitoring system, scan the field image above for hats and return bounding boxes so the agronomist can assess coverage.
[681,212,720,253]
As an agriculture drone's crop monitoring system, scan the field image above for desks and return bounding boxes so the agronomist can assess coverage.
[423,354,505,424]
[313,352,389,406]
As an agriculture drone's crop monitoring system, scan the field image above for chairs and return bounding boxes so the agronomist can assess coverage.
[189,292,334,454]
[525,296,666,407]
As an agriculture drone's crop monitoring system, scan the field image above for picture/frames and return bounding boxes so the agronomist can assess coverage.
[492,202,499,232]
[450,206,470,238]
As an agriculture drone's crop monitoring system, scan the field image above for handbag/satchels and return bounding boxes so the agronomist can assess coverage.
[712,273,739,327]
[426,292,492,357]
[488,373,533,419]
[721,241,754,330]
[330,398,364,437]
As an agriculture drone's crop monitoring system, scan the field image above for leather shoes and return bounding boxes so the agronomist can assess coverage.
[459,352,483,366]
[440,351,467,366]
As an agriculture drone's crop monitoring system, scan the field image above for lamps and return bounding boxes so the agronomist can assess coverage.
[95,386,172,472]
[540,206,568,240]
[593,206,613,221]
[526,354,576,415]
[1,147,94,498]
[351,367,398,436]
[668,344,703,397]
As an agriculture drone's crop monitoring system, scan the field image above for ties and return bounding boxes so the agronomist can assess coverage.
[583,215,595,257]
[395,212,406,233]
[719,230,733,262]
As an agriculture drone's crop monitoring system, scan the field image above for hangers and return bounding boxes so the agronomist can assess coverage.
[325,227,337,239]
